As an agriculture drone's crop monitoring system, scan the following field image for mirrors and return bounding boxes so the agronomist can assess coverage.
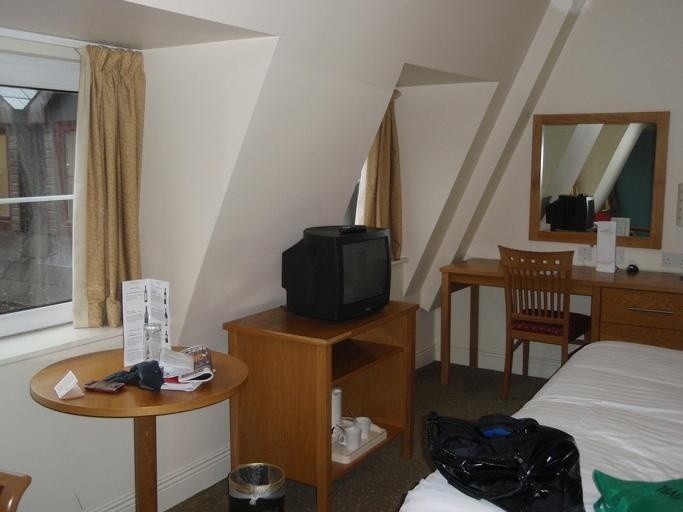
[529,111,670,249]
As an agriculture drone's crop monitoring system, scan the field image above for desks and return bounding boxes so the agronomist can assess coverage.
[439,258,683,386]
[30,347,249,512]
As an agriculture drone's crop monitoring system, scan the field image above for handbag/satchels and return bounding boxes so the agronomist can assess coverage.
[423,408,586,512]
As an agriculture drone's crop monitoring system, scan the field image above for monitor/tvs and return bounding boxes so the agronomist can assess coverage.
[283,225,392,320]
[544,194,594,231]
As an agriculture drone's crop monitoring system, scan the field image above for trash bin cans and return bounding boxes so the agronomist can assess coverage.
[228,463,285,511]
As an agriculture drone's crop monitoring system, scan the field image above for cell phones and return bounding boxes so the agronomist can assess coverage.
[83,380,125,394]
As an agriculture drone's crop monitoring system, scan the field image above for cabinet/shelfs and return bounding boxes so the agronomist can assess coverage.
[222,301,419,512]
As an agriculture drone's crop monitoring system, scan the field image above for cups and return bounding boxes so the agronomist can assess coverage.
[338,426,361,449]
[353,416,371,440]
[332,389,343,429]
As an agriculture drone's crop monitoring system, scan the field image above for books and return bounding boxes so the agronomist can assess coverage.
[157,343,215,392]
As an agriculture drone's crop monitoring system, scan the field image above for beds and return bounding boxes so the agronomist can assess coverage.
[399,341,683,512]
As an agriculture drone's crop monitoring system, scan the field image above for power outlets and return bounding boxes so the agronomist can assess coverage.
[661,252,683,267]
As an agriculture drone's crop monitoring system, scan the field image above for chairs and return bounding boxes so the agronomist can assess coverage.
[497,244,591,401]
[1,470,32,512]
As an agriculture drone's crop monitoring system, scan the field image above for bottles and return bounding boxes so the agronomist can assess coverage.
[143,285,169,360]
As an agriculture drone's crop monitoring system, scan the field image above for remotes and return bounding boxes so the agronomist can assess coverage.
[342,223,366,232]
